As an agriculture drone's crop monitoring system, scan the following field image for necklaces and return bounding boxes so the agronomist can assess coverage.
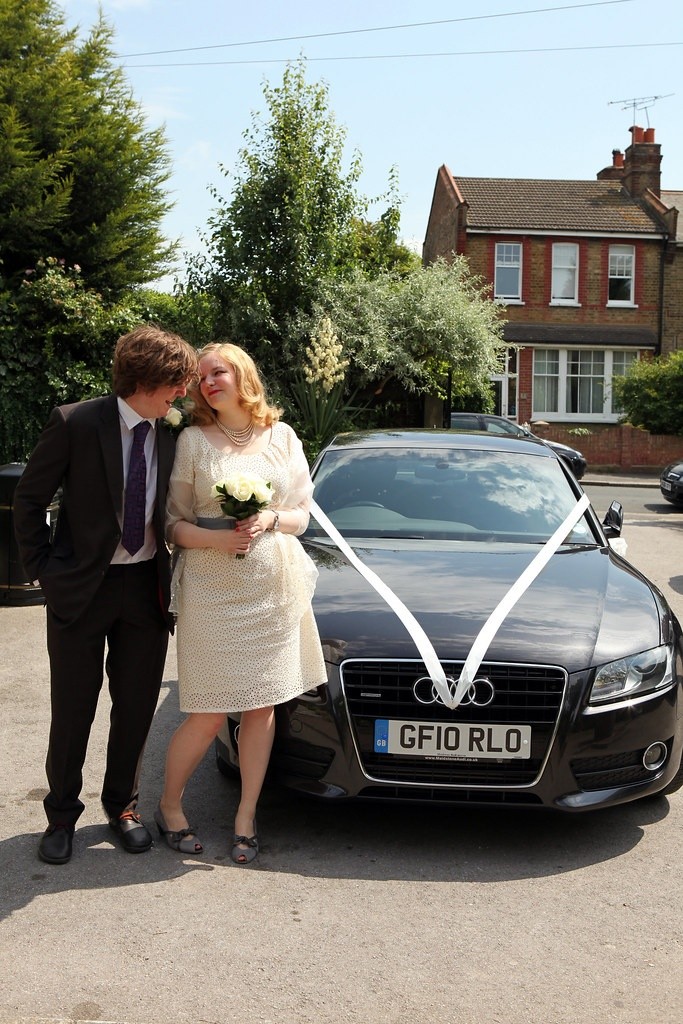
[217,420,256,446]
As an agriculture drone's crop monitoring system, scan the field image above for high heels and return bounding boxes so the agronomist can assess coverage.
[153,800,204,854]
[231,816,259,863]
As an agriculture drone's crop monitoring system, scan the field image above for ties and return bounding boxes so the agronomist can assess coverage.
[120,421,152,556]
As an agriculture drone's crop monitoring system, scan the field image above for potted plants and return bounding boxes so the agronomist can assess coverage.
[625,421,647,438]
[567,429,594,443]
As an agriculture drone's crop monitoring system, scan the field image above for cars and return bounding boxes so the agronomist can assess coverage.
[450,411,588,480]
[658,459,683,510]
[216,426,683,817]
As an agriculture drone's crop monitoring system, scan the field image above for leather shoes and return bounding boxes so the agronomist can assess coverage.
[109,808,152,853]
[38,823,74,864]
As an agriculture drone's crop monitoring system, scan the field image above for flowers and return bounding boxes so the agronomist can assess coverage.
[210,472,276,560]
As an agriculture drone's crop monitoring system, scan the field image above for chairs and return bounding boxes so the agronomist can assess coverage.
[336,455,398,509]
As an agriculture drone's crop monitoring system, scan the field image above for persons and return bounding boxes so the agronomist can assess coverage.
[153,341,328,862]
[13,323,200,864]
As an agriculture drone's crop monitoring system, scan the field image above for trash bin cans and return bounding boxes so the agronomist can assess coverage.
[0,462,64,607]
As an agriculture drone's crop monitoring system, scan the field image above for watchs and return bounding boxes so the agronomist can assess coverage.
[266,509,280,532]
